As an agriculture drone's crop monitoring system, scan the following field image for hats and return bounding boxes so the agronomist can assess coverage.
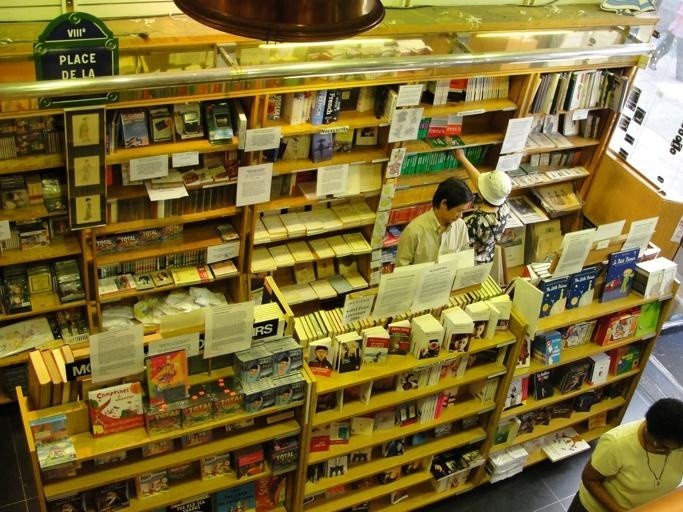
[477,170,511,206]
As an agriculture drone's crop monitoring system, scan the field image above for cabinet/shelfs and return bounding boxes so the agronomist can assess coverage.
[2,7,680,510]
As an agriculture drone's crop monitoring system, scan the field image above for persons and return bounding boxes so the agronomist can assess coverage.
[567,398,683,512]
